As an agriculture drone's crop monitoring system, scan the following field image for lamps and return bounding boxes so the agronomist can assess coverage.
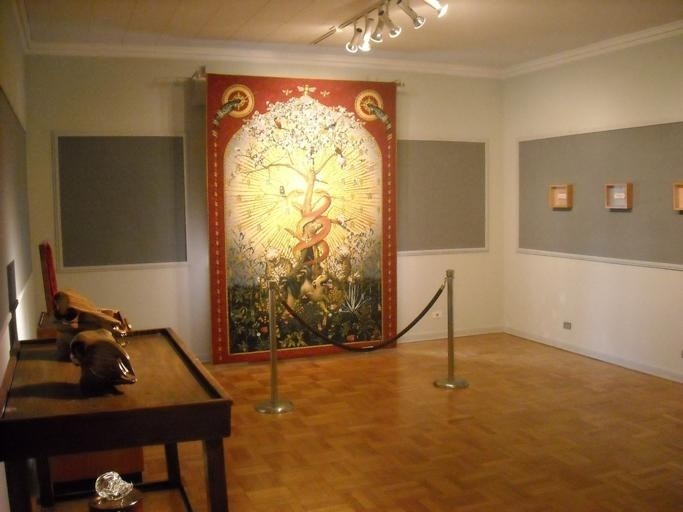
[344,1,450,55]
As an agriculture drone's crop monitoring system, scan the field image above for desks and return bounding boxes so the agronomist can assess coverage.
[0,328,232,510]
